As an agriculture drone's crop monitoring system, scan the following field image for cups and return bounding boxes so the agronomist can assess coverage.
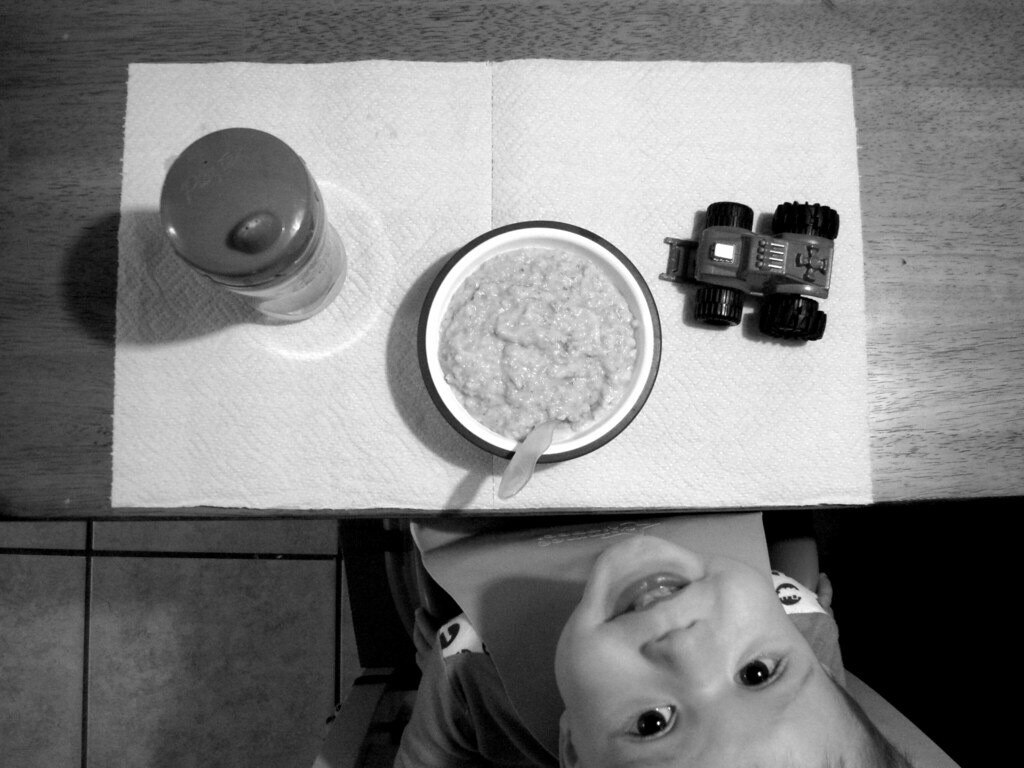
[159,126,348,322]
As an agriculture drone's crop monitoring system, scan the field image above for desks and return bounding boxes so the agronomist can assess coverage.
[0,2,1024,520]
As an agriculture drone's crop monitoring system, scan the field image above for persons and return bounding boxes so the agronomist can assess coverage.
[375,512,918,768]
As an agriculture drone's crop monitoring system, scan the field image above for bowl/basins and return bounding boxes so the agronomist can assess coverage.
[416,219,666,466]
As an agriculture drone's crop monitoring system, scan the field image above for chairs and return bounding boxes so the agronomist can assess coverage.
[314,520,962,768]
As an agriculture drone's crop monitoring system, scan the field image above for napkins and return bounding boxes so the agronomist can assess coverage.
[110,59,874,511]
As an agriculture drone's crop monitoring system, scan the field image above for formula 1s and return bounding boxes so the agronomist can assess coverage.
[657,199,842,342]
